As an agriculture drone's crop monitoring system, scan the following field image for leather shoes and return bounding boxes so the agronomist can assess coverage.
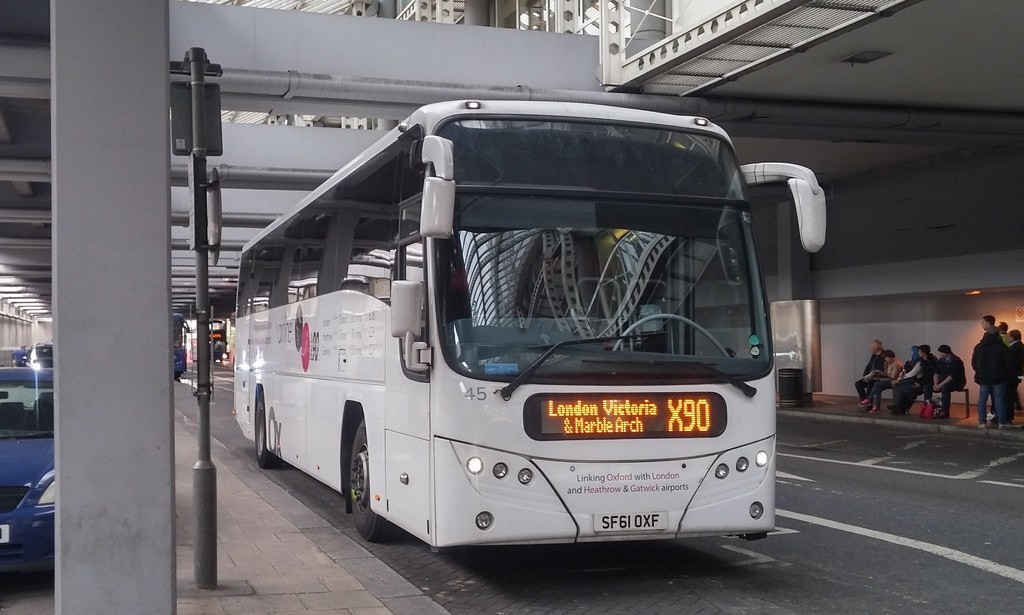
[887,404,909,416]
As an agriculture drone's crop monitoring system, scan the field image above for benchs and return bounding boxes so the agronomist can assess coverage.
[883,385,970,419]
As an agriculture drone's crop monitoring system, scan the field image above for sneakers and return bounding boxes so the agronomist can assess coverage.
[977,423,988,430]
[870,405,880,413]
[857,399,871,407]
[997,423,1018,429]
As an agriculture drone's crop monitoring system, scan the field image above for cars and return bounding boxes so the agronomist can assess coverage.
[27,342,53,367]
[0,365,55,580]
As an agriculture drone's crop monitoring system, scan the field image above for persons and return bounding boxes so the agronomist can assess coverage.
[985,320,1013,422]
[924,345,966,419]
[981,315,1013,425]
[885,344,921,413]
[891,344,940,415]
[1005,328,1024,423]
[971,325,1017,431]
[854,339,889,407]
[858,349,903,411]
[11,344,28,368]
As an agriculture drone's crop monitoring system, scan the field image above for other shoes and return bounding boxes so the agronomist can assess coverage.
[921,400,949,419]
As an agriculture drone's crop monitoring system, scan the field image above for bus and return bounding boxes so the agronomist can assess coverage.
[233,99,826,552]
[188,319,227,363]
[173,312,190,378]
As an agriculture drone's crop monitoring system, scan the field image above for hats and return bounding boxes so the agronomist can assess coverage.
[938,345,952,353]
[987,325,1000,336]
